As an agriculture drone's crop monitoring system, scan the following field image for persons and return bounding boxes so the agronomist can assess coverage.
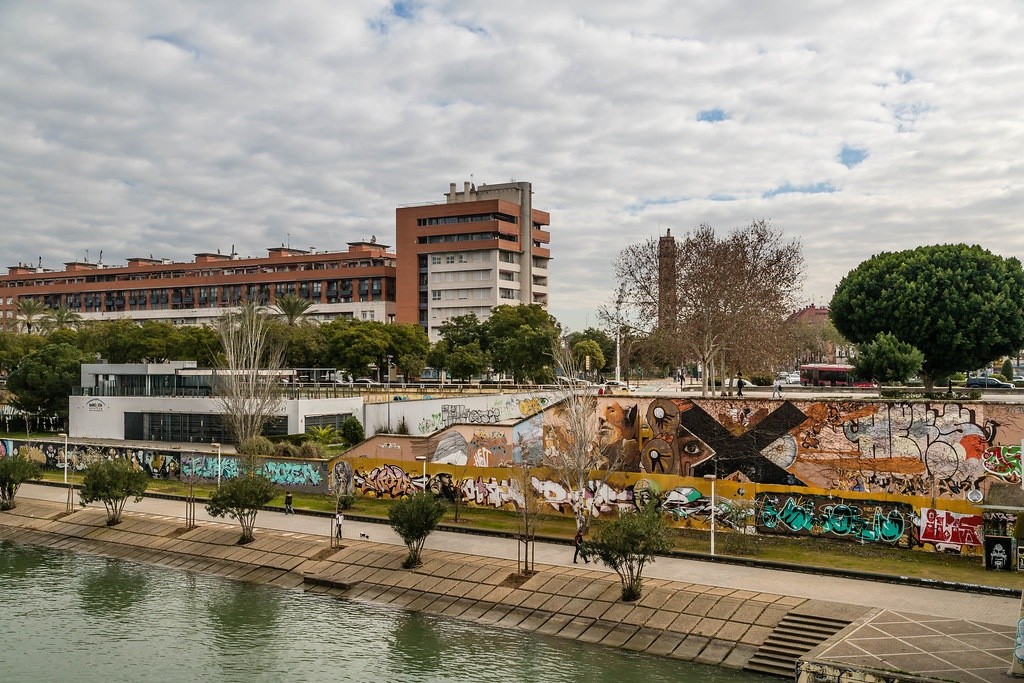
[786,374,790,386]
[336,511,344,540]
[284,490,295,515]
[573,529,590,564]
[598,385,613,395]
[598,374,606,385]
[673,371,685,385]
[737,376,746,396]
[771,377,782,399]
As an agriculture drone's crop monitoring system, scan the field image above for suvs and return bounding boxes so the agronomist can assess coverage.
[965,376,1015,388]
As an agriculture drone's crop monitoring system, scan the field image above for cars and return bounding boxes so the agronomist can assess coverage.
[554,375,592,389]
[778,372,789,380]
[785,373,800,384]
[1012,375,1024,381]
[278,376,384,388]
[598,379,636,393]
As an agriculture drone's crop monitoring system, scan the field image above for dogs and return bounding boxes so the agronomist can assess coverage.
[360,532,369,539]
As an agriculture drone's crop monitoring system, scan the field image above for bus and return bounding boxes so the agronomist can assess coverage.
[800,363,878,390]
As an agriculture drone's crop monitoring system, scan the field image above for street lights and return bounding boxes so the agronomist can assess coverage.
[210,444,222,492]
[703,474,716,555]
[57,433,68,484]
[617,325,625,381]
[385,364,394,433]
[415,456,427,496]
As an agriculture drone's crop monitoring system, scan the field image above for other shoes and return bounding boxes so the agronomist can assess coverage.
[340,538,342,540]
[293,512,294,515]
[574,562,577,563]
[586,561,590,564]
[285,513,287,515]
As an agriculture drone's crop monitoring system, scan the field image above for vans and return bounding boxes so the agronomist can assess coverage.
[724,378,758,388]
[792,371,800,375]
